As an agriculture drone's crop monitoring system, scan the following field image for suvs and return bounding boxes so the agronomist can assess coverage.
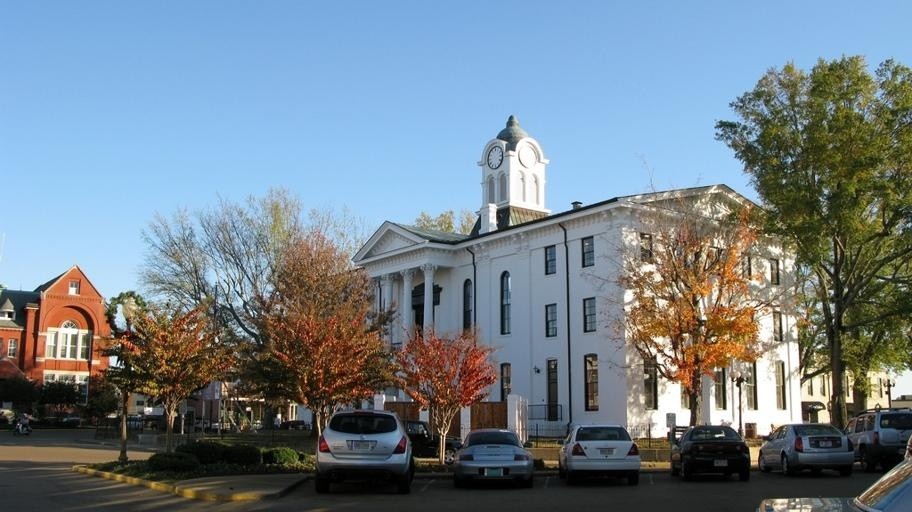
[844,404,912,472]
[403,420,464,465]
[314,409,416,496]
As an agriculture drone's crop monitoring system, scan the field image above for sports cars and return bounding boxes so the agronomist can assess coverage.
[452,427,536,489]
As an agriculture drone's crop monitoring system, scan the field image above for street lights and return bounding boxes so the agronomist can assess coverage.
[882,374,897,409]
[730,366,752,438]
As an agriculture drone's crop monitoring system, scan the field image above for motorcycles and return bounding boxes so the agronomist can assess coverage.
[12,420,32,437]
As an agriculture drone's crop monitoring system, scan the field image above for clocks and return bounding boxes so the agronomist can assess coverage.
[518,144,538,168]
[486,144,503,171]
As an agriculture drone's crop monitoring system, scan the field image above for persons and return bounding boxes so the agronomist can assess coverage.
[273,414,280,432]
[18,412,30,431]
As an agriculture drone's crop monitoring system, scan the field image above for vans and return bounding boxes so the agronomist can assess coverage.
[62,417,80,427]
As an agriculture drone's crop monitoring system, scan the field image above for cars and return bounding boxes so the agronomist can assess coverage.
[757,422,856,477]
[557,424,642,487]
[669,425,752,483]
[755,453,912,512]
[193,419,222,432]
[124,415,143,429]
